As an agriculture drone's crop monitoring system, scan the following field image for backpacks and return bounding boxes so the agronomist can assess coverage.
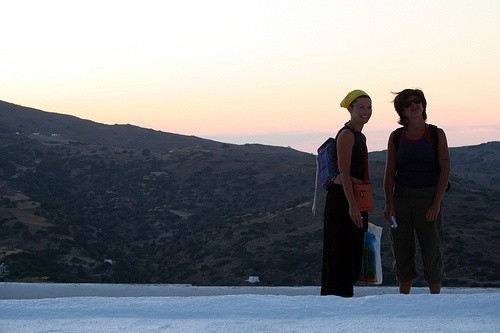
[317,126,356,188]
[354,221,383,285]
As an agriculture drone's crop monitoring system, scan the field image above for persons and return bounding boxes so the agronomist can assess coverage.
[384,89,451,293]
[320,90,373,298]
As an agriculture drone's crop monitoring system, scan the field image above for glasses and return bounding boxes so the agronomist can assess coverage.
[401,98,422,108]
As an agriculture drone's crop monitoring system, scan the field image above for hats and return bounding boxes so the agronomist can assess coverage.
[340,90,370,109]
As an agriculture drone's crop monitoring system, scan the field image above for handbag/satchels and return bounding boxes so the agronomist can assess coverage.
[353,182,373,212]
